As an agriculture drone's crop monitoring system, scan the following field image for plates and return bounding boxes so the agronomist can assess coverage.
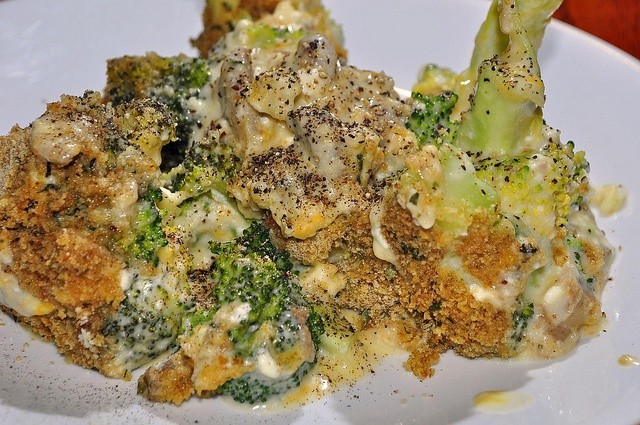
[1,0,639,424]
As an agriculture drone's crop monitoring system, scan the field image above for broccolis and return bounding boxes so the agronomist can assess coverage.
[181,219,326,404]
[406,60,593,260]
